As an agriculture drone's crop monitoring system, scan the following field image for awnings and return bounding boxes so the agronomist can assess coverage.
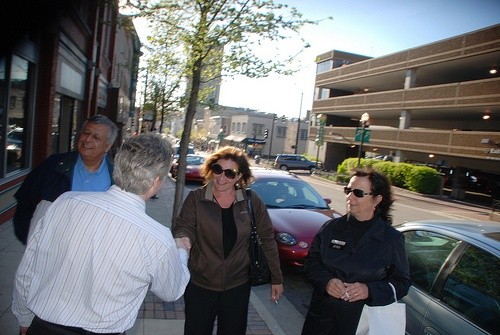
[224,133,246,142]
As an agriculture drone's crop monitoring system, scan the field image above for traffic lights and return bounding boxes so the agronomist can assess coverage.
[273,113,277,120]
[282,116,286,122]
[264,128,268,138]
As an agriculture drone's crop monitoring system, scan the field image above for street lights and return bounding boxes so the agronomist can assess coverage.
[316,113,326,161]
[358,113,370,165]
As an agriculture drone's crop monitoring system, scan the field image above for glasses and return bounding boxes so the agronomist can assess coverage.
[344,187,379,197]
[209,163,240,179]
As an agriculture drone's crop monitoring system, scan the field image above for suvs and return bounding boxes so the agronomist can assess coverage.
[274,154,322,173]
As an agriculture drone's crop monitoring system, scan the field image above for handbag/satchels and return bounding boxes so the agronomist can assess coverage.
[355,282,406,335]
[246,188,272,285]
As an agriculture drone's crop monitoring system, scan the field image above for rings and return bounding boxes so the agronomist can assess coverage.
[355,292,358,297]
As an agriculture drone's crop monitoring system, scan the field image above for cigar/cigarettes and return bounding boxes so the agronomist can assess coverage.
[346,292,350,298]
[276,301,279,304]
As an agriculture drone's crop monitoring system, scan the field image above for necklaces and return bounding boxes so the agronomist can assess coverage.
[213,194,235,208]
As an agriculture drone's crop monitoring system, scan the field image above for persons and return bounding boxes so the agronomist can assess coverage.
[11,130,191,335]
[300,165,412,335]
[13,114,119,245]
[172,146,285,335]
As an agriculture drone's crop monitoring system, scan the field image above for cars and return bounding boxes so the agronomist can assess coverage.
[237,169,343,270]
[170,139,204,182]
[392,221,500,335]
